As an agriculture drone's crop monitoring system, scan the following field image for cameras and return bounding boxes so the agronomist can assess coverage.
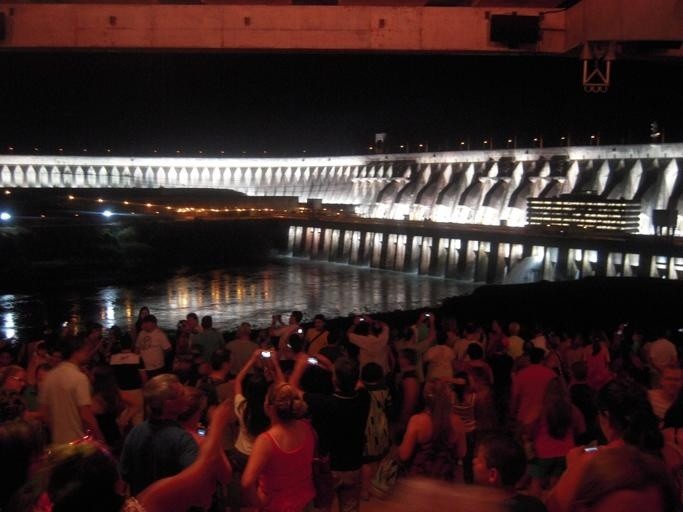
[261,351,271,358]
[424,312,430,318]
[359,317,365,322]
[307,357,318,365]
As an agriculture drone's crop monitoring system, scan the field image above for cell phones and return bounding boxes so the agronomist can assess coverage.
[197,428,206,437]
[296,327,304,335]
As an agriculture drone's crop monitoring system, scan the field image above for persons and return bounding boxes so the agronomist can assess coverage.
[1,307,682,511]
